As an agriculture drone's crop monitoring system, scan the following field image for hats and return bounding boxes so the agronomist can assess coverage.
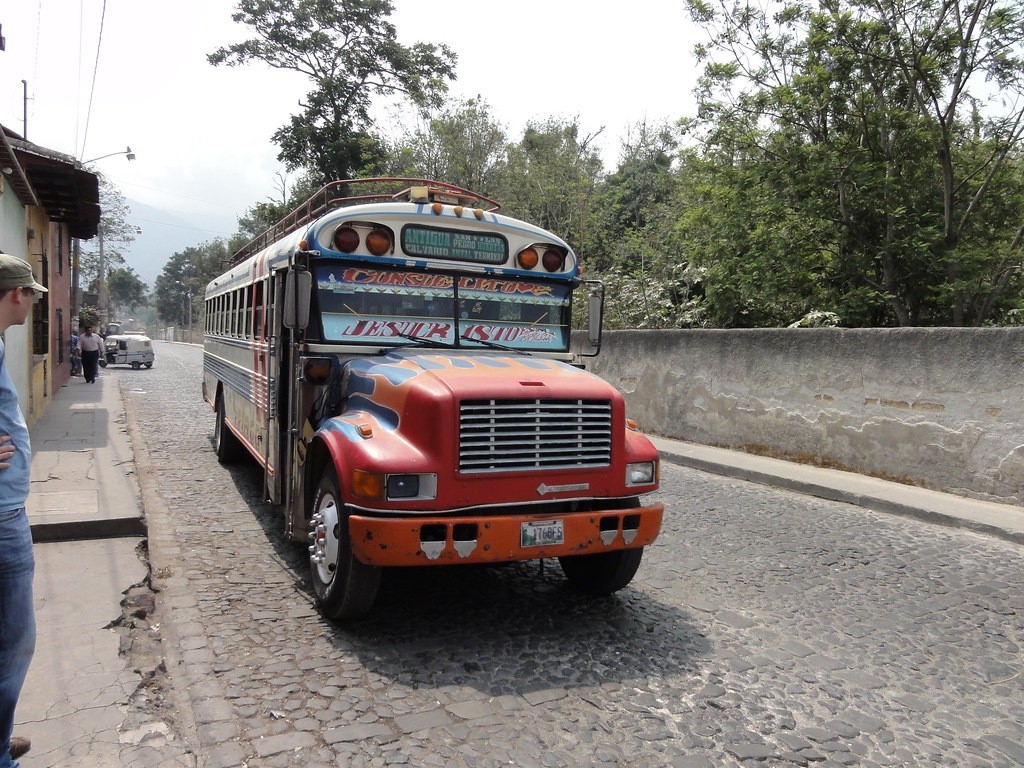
[0,255,48,291]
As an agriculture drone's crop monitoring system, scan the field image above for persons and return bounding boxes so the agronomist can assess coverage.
[0,253,48,768]
[70,327,105,384]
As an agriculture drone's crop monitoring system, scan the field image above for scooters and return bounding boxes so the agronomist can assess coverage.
[97,335,155,370]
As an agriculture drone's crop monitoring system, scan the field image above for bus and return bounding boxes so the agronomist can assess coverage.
[202,177,667,623]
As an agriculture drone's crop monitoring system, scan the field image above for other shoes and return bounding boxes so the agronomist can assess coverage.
[10,736,31,760]
